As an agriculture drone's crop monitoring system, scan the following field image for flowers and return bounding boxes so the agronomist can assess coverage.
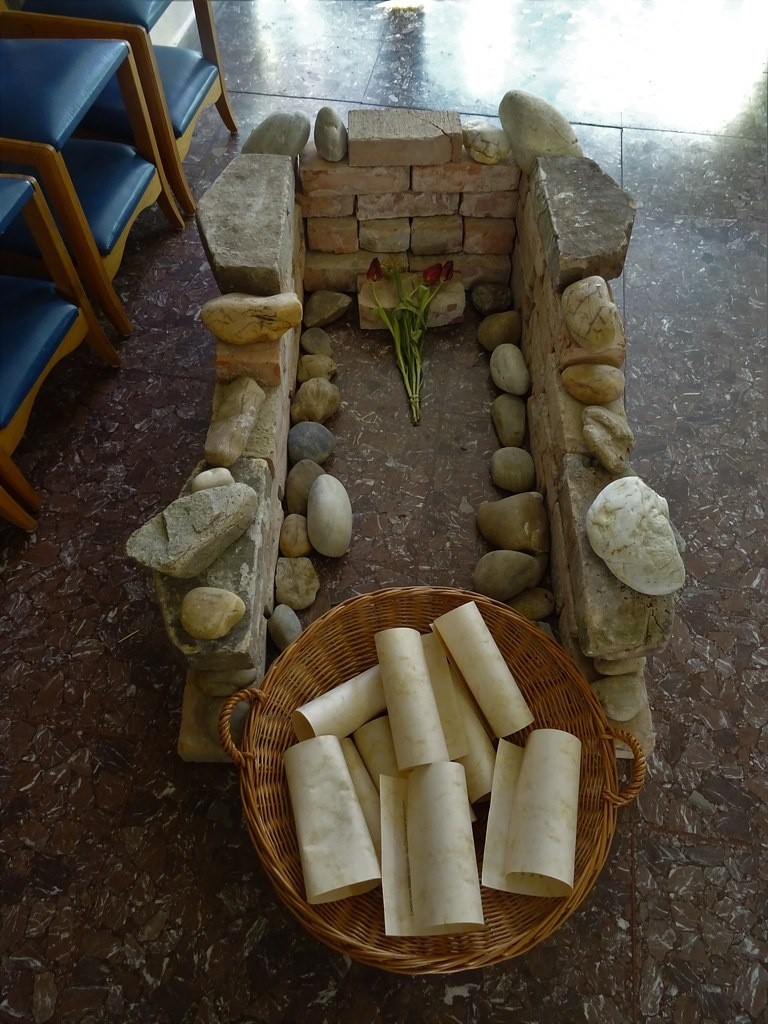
[366,256,454,424]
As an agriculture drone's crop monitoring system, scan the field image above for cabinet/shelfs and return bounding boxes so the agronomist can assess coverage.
[0,38,184,336]
[0,0,240,214]
[0,173,119,509]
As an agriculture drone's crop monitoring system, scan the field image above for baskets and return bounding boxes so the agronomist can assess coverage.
[219,585,648,979]
